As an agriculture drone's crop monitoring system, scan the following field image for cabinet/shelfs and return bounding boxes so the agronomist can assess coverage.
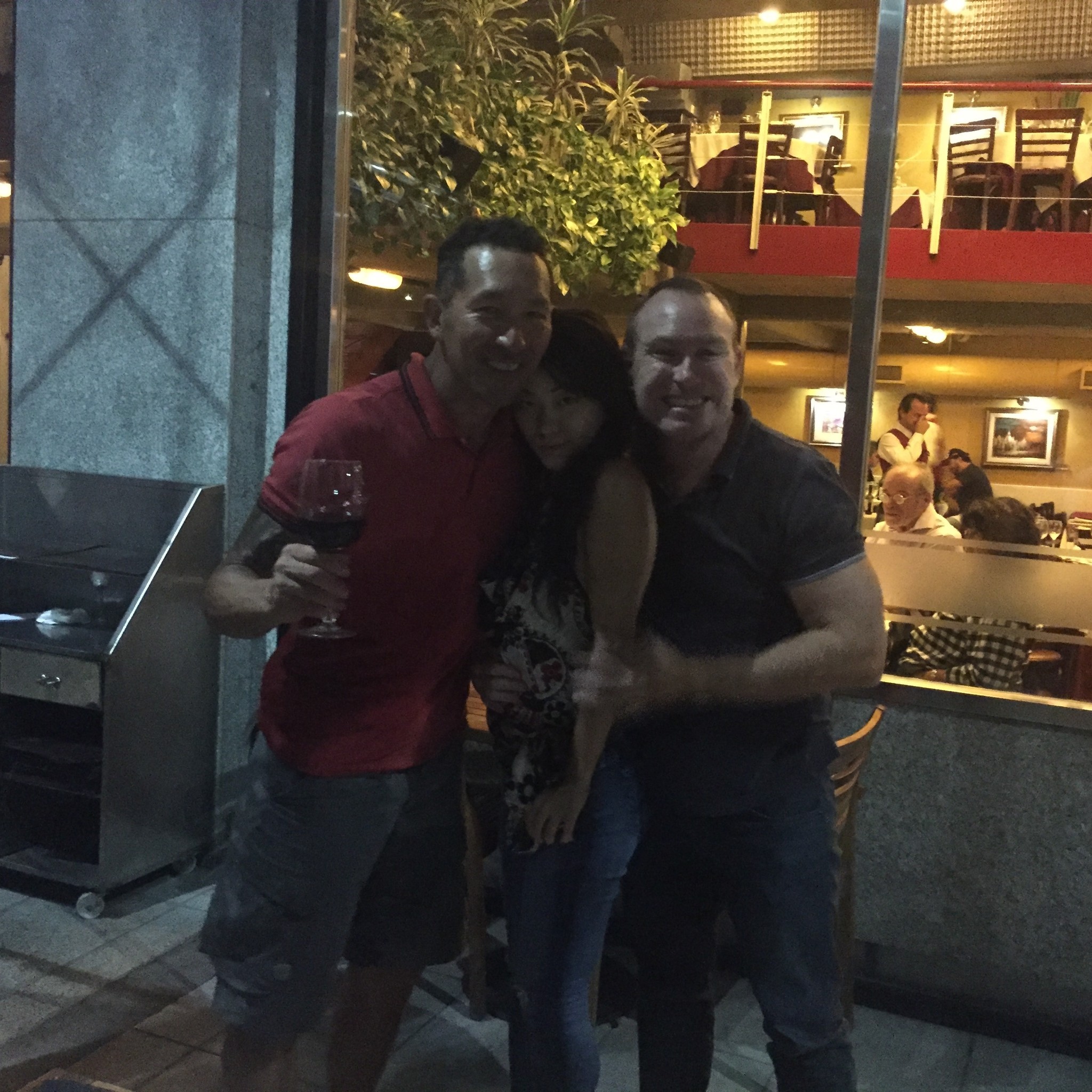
[0,465,227,921]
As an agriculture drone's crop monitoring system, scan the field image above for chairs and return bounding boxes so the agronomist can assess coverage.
[1030,501,1055,520]
[932,107,1092,232]
[828,704,887,1035]
[650,122,846,226]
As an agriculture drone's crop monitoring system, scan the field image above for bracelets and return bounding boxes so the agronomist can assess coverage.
[930,668,937,680]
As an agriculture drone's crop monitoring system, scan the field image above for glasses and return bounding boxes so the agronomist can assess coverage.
[879,490,925,505]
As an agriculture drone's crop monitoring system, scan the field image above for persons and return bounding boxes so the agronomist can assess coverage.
[865,392,1039,692]
[203,217,885,1088]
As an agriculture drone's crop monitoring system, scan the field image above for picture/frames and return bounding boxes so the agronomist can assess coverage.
[981,407,1061,472]
[779,110,849,170]
[808,396,846,448]
[931,101,1013,153]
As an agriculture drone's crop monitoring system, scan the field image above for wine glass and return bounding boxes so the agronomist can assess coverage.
[298,458,369,640]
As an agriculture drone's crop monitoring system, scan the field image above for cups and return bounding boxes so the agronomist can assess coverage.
[1022,118,1086,132]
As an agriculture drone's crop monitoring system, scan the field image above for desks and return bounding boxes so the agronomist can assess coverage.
[657,133,831,194]
[1070,512,1092,520]
[935,131,1092,213]
[826,187,923,229]
[461,688,600,1026]
